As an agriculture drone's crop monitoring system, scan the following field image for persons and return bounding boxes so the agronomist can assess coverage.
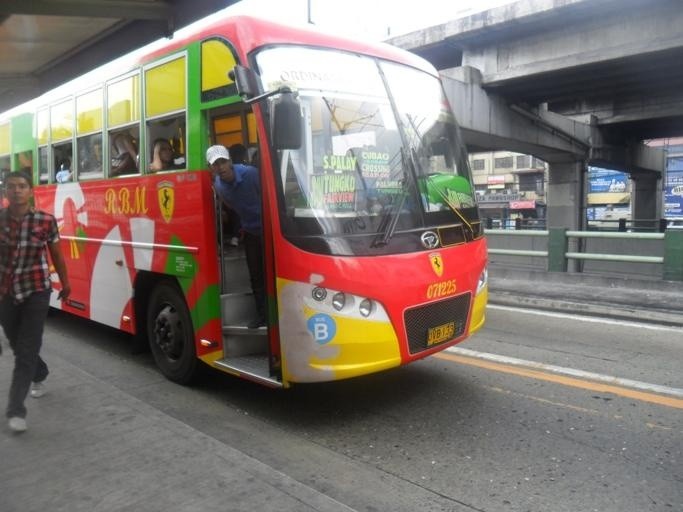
[205,144,268,329]
[0,171,71,433]
[0,132,174,190]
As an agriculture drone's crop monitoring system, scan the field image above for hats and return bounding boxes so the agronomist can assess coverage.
[206,144,230,165]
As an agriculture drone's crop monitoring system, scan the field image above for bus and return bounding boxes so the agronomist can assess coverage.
[0,17,489,394]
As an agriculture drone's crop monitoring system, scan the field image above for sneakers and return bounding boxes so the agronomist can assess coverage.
[30,376,48,398]
[9,417,26,432]
[230,237,239,247]
[248,315,267,328]
